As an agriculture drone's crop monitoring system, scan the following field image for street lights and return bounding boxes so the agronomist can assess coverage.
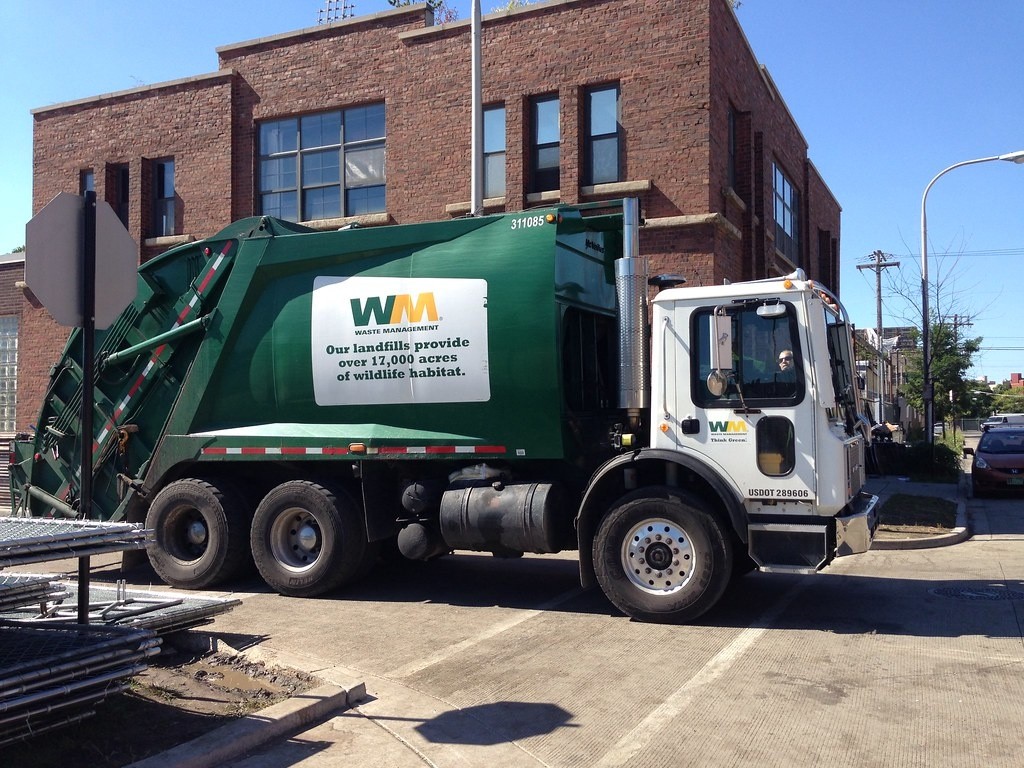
[920,148,1024,468]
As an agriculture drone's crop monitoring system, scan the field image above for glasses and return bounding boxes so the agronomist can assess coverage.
[776,356,793,365]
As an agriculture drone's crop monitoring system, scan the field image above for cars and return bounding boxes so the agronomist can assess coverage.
[963,428,1024,494]
[981,414,1024,434]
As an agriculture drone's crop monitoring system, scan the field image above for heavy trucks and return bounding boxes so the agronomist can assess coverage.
[8,196,880,623]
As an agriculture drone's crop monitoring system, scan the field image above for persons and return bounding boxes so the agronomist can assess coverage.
[766,350,796,383]
